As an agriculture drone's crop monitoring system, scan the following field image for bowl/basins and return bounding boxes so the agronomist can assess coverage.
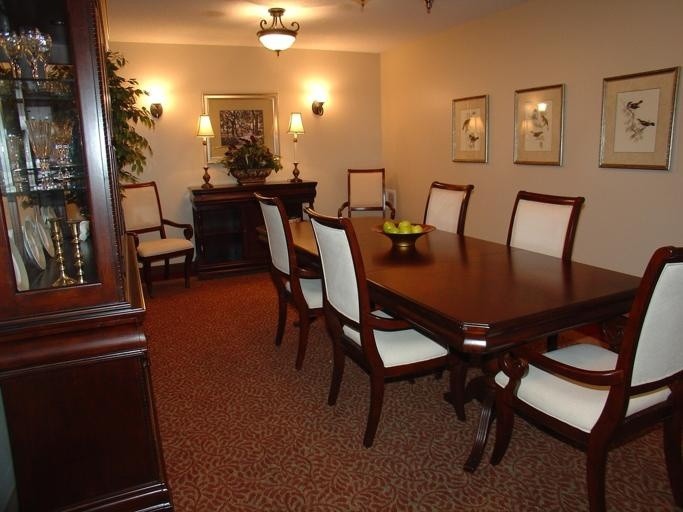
[369,223,436,247]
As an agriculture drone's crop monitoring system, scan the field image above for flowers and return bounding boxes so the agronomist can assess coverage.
[219,134,283,174]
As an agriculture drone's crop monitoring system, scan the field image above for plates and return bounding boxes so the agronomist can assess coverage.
[7,206,62,293]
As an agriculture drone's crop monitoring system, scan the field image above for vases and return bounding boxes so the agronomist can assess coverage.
[232,169,270,186]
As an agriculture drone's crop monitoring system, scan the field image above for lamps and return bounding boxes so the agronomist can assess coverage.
[196,114,215,190]
[256,7,299,58]
[305,79,328,116]
[285,112,304,185]
[146,81,166,120]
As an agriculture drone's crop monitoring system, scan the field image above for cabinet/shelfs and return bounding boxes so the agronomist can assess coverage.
[186,179,318,279]
[0,1,173,511]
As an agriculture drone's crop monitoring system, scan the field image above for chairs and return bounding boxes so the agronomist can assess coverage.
[117,182,194,299]
[421,180,475,234]
[252,190,375,371]
[336,166,396,220]
[491,246,682,512]
[301,204,472,449]
[473,188,584,355]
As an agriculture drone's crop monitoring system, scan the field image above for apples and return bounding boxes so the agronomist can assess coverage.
[383,220,423,234]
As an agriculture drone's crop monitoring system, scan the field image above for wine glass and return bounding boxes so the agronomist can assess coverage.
[0,27,53,97]
[4,115,77,190]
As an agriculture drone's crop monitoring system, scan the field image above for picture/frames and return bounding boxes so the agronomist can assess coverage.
[451,93,489,164]
[512,82,566,168]
[202,91,280,167]
[597,65,680,170]
